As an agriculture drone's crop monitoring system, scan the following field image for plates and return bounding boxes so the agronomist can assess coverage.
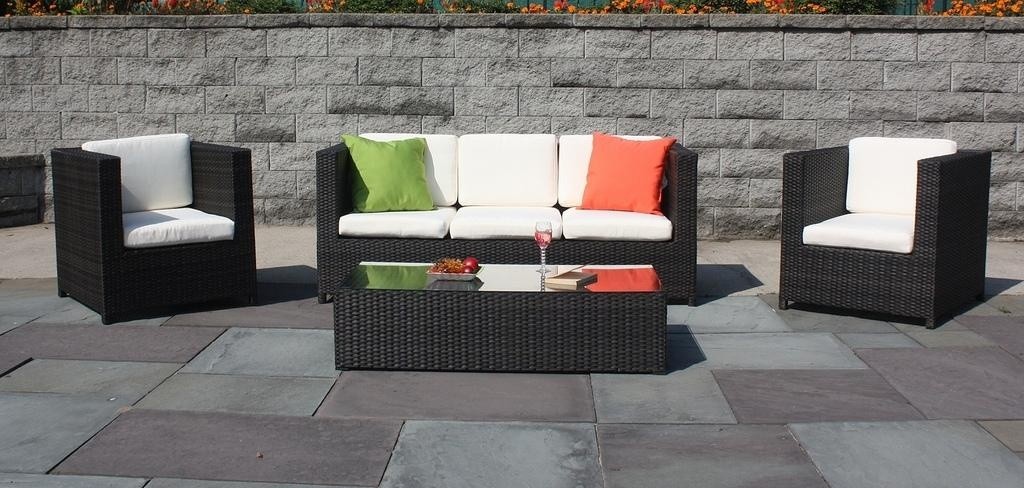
[424,265,485,280]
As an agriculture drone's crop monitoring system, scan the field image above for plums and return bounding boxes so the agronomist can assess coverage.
[461,257,479,274]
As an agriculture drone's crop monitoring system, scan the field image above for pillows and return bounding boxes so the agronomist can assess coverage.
[576,130,677,217]
[339,134,438,212]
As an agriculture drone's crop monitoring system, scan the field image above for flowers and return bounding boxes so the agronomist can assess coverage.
[0,0,1023,16]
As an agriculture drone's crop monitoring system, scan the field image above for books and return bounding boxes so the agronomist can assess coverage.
[545,265,598,286]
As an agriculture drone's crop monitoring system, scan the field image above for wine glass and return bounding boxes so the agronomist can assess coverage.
[535,222,552,274]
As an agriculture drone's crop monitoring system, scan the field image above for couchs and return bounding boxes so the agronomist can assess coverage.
[315,131,699,306]
[778,137,992,329]
[49,133,257,325]
